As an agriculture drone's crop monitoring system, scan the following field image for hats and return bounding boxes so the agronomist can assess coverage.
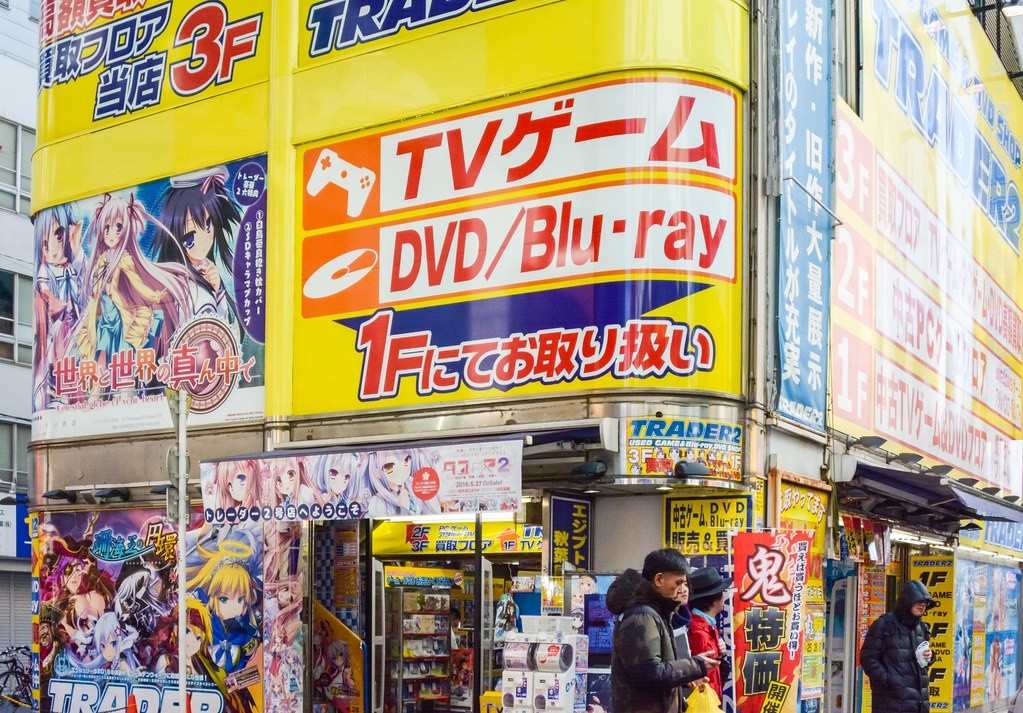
[685,566,733,600]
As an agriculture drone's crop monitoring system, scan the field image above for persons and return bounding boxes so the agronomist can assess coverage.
[688,566,732,709]
[990,640,1002,700]
[860,581,937,713]
[672,572,694,704]
[450,607,461,650]
[606,548,721,713]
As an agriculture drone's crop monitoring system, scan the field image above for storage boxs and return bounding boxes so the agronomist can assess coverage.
[520,615,576,633]
[501,633,590,713]
[860,572,886,649]
[377,593,450,713]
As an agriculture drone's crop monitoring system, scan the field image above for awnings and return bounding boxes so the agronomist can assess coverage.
[857,462,1023,525]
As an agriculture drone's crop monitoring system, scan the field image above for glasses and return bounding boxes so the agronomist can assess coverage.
[917,600,930,606]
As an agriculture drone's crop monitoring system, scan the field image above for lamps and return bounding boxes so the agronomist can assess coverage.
[845,434,887,450]
[1003,495,1020,503]
[674,461,711,480]
[569,460,607,476]
[919,465,954,475]
[957,478,980,487]
[885,450,924,465]
[976,487,1001,496]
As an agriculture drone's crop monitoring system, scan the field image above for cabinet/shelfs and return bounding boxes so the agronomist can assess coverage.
[376,588,452,713]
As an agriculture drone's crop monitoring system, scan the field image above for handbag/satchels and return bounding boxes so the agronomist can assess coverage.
[685,683,725,713]
